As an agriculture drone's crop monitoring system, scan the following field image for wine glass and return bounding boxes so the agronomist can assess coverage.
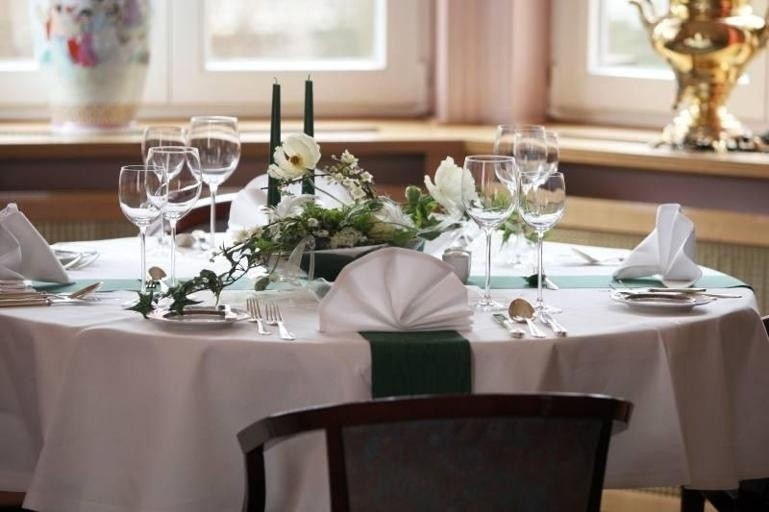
[118,116,241,307]
[461,124,565,314]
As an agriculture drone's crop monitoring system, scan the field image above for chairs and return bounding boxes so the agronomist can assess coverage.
[237,392,634,512]
[680,315,769,512]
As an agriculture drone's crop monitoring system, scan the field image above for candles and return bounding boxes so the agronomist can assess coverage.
[302,74,315,194]
[268,76,281,207]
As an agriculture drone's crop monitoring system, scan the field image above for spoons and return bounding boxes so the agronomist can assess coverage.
[509,298,545,338]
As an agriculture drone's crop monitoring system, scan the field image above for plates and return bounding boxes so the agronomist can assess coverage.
[609,287,716,311]
[146,305,250,327]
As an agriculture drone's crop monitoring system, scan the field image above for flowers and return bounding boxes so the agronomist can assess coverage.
[124,134,559,321]
[612,204,703,293]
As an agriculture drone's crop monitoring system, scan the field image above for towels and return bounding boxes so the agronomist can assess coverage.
[0,202,76,292]
[316,247,477,335]
[227,167,359,239]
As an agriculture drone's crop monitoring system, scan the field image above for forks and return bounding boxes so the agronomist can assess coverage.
[537,310,567,334]
[608,283,743,302]
[40,281,103,303]
[52,246,99,270]
[248,299,295,340]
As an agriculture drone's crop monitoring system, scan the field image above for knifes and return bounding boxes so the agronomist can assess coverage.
[218,304,237,319]
[491,313,526,336]
